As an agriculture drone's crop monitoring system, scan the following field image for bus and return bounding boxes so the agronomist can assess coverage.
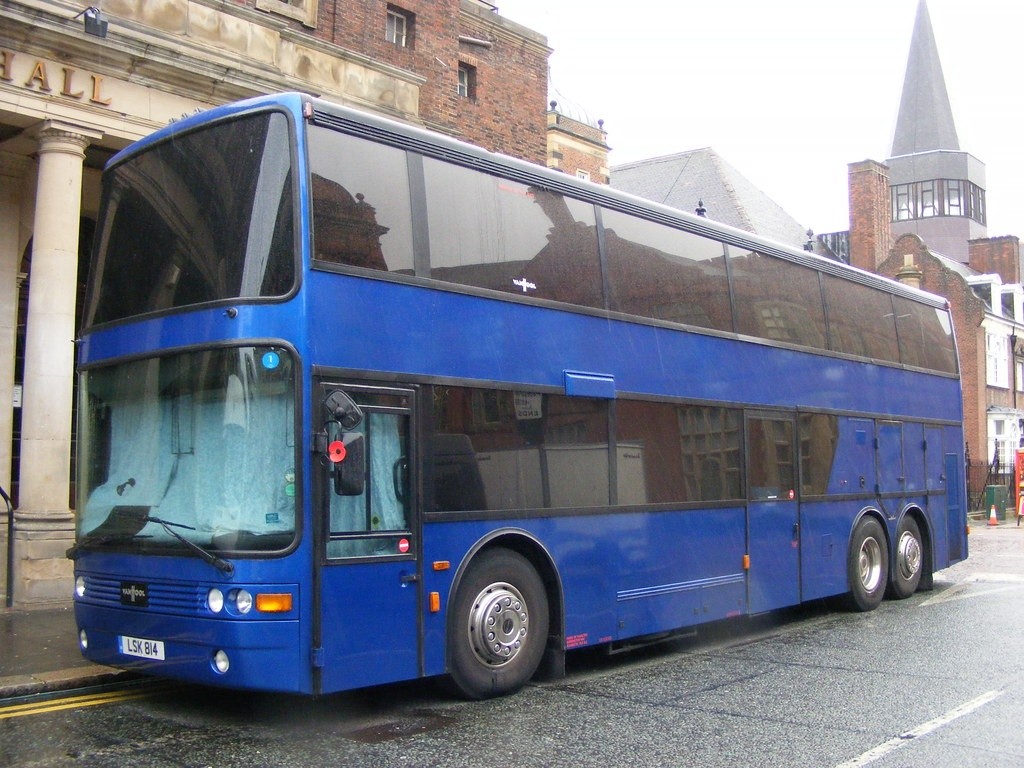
[65,92,969,705]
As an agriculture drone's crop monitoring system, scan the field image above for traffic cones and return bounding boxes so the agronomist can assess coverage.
[987,504,1000,526]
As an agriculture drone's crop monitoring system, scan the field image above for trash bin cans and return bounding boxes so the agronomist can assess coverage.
[985,485,1007,522]
